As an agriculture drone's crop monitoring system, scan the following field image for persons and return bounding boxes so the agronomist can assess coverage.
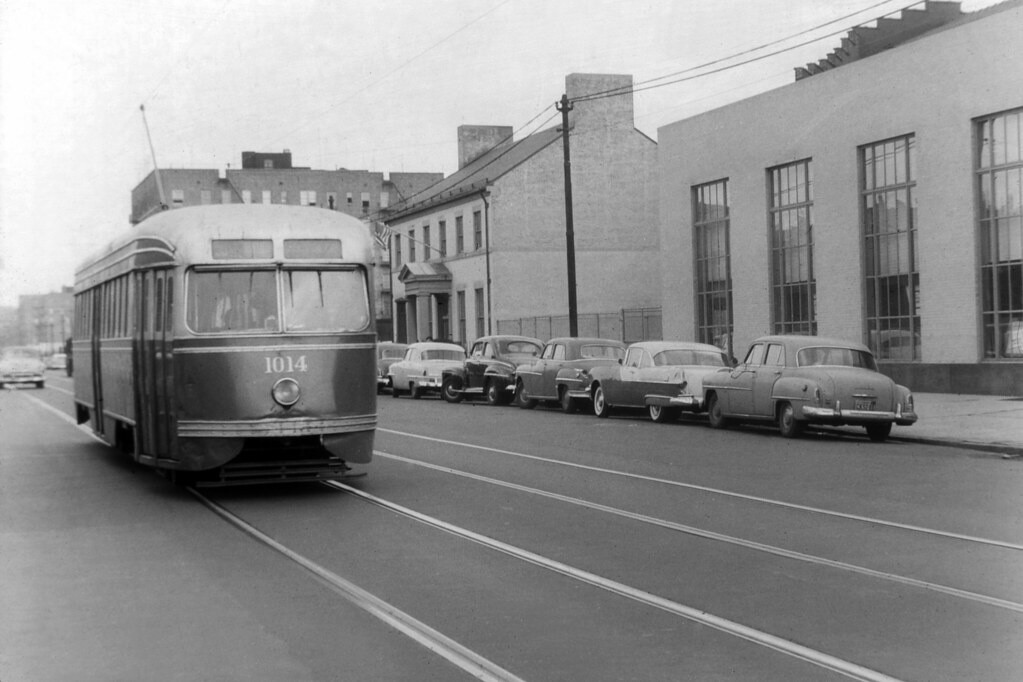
[211,271,259,332]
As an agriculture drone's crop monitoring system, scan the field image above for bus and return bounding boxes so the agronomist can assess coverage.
[71,204,378,491]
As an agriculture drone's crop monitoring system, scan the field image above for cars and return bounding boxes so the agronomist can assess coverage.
[377,341,410,389]
[386,341,467,399]
[438,334,546,406]
[586,340,732,423]
[1,347,44,388]
[698,334,917,443]
[514,337,626,412]
